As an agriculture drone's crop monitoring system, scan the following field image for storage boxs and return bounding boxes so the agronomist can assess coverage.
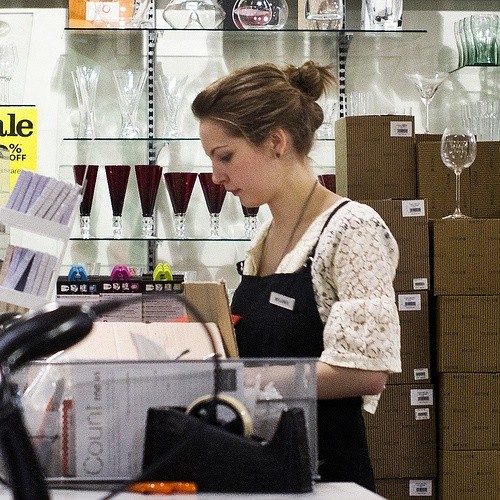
[374,476,437,500]
[334,114,418,201]
[385,292,434,385]
[354,198,432,292]
[416,140,500,500]
[361,381,437,479]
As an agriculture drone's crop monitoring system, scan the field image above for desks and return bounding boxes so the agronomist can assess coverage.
[0,473,389,500]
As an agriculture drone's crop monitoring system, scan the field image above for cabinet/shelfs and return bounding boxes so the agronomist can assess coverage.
[64,26,500,243]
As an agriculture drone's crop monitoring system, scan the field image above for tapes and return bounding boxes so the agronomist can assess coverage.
[184,394,255,438]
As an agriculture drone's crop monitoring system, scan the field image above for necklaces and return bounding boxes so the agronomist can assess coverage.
[280,179,318,263]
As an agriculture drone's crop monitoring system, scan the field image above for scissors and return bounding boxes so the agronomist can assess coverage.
[113,478,199,494]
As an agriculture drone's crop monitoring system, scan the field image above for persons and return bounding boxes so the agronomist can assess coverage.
[192,59,402,493]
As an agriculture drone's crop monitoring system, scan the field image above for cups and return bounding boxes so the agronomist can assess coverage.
[94,0,150,27]
[453,13,500,66]
[314,91,375,138]
[461,101,500,142]
[164,0,405,31]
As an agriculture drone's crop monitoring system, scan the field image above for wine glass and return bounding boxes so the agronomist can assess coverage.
[72,61,189,140]
[135,164,165,240]
[403,69,449,133]
[440,126,478,221]
[241,201,259,241]
[164,172,198,239]
[199,171,227,240]
[104,164,133,241]
[72,164,100,240]
